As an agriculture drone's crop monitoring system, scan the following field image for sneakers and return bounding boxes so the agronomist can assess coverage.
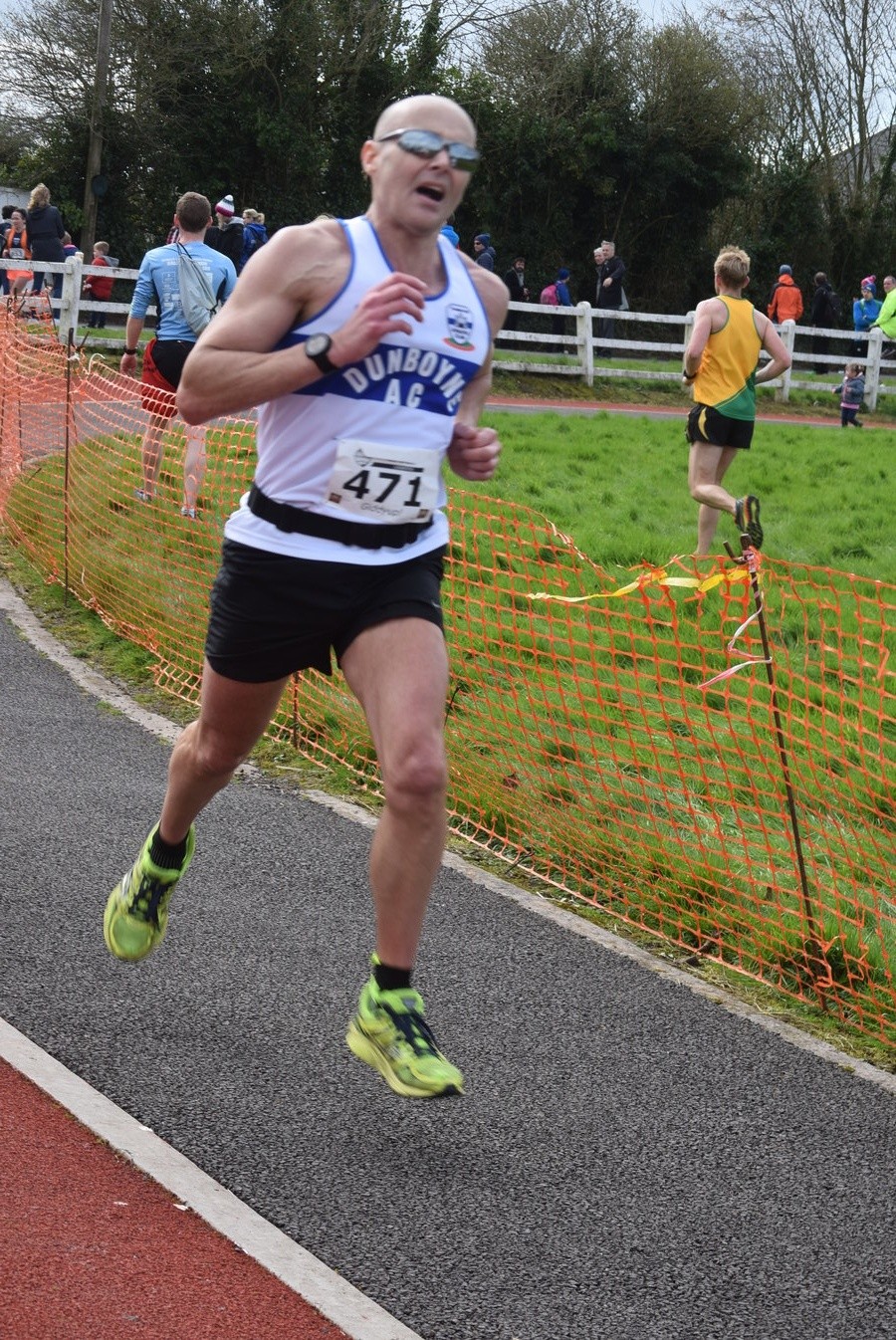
[104,817,197,963]
[344,953,468,1099]
[734,494,764,552]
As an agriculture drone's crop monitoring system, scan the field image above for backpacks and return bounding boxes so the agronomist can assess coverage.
[540,284,558,306]
[822,287,844,324]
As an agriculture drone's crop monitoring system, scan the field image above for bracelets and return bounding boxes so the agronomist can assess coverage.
[683,369,696,379]
[124,344,137,355]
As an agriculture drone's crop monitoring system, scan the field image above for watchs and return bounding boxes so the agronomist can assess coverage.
[303,333,339,378]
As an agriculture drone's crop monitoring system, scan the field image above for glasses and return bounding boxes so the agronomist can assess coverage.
[377,127,484,175]
[473,241,481,246]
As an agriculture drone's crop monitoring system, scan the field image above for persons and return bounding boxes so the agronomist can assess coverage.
[811,273,834,375]
[593,240,626,358]
[766,265,804,325]
[0,181,81,327]
[832,362,866,426]
[473,233,497,272]
[121,191,236,524]
[545,269,572,353]
[161,196,267,276]
[504,257,530,348]
[439,211,460,252]
[850,273,896,373]
[684,244,793,557]
[82,242,119,330]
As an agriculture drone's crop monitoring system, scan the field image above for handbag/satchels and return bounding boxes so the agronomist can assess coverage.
[244,226,264,257]
[177,252,220,332]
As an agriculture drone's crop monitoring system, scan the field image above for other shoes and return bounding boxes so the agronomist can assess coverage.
[881,347,895,356]
[135,487,156,503]
[181,507,196,521]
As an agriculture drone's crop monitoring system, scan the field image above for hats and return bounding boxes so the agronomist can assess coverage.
[557,268,569,280]
[779,264,793,277]
[864,282,877,298]
[475,232,491,248]
[861,274,876,287]
[215,194,235,217]
